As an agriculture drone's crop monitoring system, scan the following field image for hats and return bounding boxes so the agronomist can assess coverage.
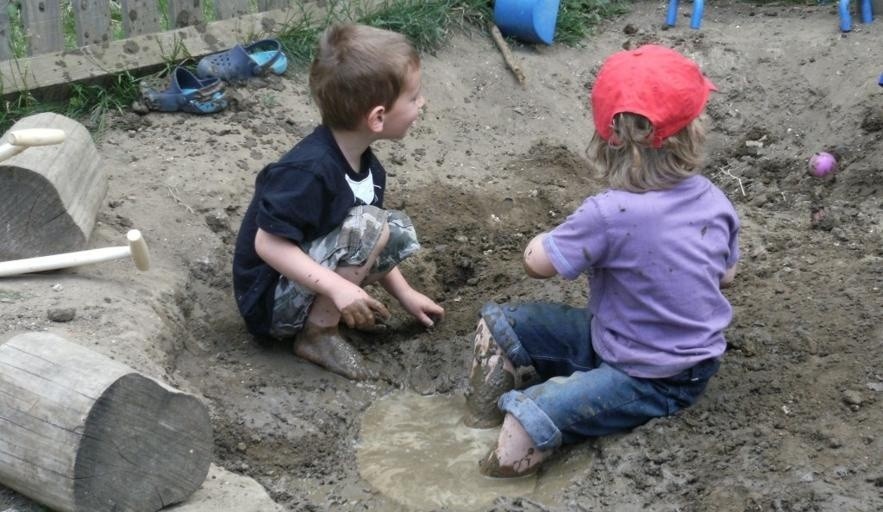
[591,44,718,148]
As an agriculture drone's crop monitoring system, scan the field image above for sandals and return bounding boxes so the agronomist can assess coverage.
[198,39,287,78]
[141,70,229,113]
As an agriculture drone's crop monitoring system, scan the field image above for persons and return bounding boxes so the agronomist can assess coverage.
[232,24,445,382]
[465,44,737,479]
